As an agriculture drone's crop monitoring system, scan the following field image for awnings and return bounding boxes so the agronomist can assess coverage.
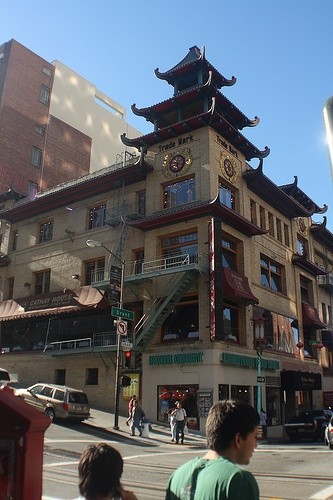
[303,302,326,329]
[0,284,108,321]
[223,268,259,306]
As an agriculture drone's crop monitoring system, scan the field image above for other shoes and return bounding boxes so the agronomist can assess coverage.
[169,439,174,442]
[174,441,183,444]
[124,420,129,426]
[129,434,142,436]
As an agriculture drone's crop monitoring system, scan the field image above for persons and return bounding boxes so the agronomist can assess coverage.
[260,408,267,440]
[165,399,260,500]
[126,395,138,426]
[78,442,138,500]
[169,401,187,444]
[131,400,146,436]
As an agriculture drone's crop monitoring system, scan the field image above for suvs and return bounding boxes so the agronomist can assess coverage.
[14,382,91,423]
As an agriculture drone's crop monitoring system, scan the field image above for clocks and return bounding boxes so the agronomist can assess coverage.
[224,159,234,177]
[169,154,186,173]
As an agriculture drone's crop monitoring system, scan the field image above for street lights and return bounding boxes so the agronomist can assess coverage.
[85,239,129,430]
[250,306,266,439]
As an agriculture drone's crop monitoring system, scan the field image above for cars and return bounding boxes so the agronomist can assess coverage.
[286,410,333,450]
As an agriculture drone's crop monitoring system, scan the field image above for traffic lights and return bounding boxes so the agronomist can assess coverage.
[135,352,141,369]
[125,351,131,368]
